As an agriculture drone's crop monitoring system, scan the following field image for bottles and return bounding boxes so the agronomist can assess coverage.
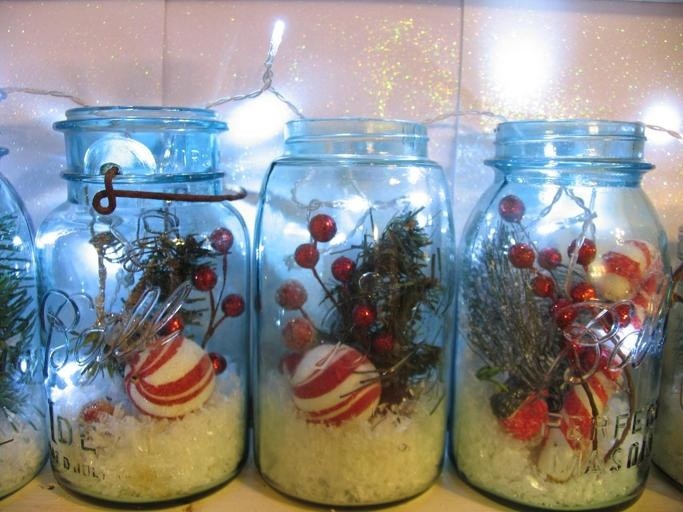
[250,115,457,510]
[447,119,675,512]
[0,89,48,504]
[47,104,253,511]
[0,290,1,295]
[648,229,683,497]
